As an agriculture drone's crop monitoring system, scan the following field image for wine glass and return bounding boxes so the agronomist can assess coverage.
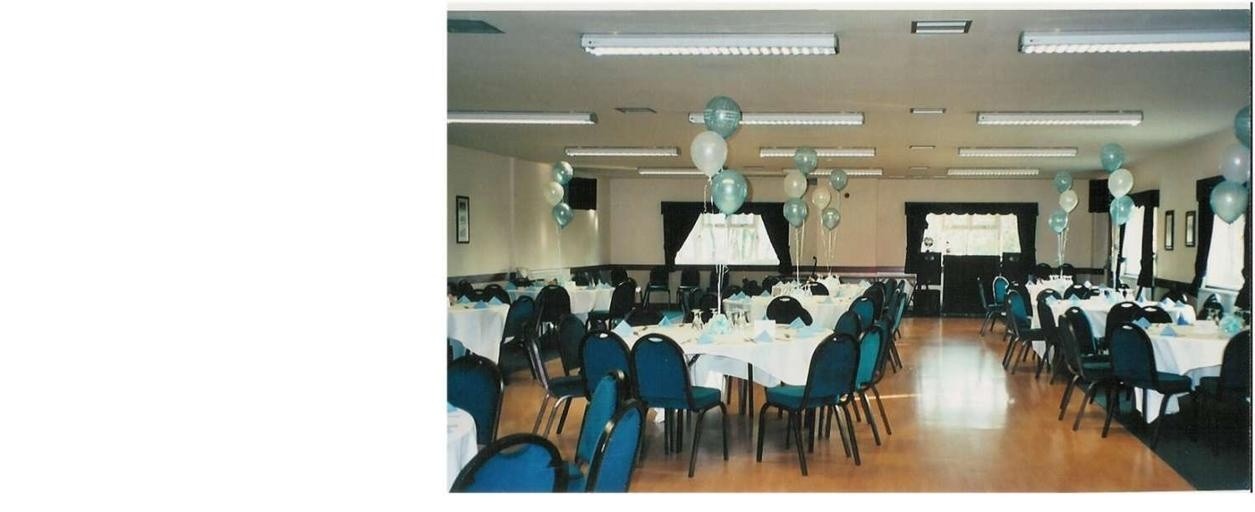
[779,282,812,302]
[1204,307,1246,335]
[1097,287,1135,308]
[816,272,840,288]
[1047,274,1073,291]
[687,306,754,345]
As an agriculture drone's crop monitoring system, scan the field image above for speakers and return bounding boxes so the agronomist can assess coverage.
[567,177,598,210]
[1089,179,1115,212]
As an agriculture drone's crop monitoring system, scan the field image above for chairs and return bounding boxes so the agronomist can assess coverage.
[449,267,916,493]
[977,264,1252,447]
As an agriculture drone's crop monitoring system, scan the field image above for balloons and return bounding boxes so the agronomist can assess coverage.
[1208,104,1251,225]
[690,96,749,215]
[543,160,574,230]
[1099,142,1134,226]
[1047,171,1079,233]
[780,145,848,232]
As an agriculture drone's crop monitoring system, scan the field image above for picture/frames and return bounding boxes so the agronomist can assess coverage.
[1164,210,1174,250]
[455,195,470,243]
[1184,210,1195,247]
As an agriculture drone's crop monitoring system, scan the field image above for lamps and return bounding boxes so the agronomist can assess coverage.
[760,147,878,158]
[564,146,684,161]
[574,34,842,59]
[638,168,717,176]
[974,109,1143,129]
[958,146,1077,159]
[448,111,597,127]
[689,113,867,127]
[782,167,886,178]
[1016,32,1252,55]
[948,168,1040,177]
